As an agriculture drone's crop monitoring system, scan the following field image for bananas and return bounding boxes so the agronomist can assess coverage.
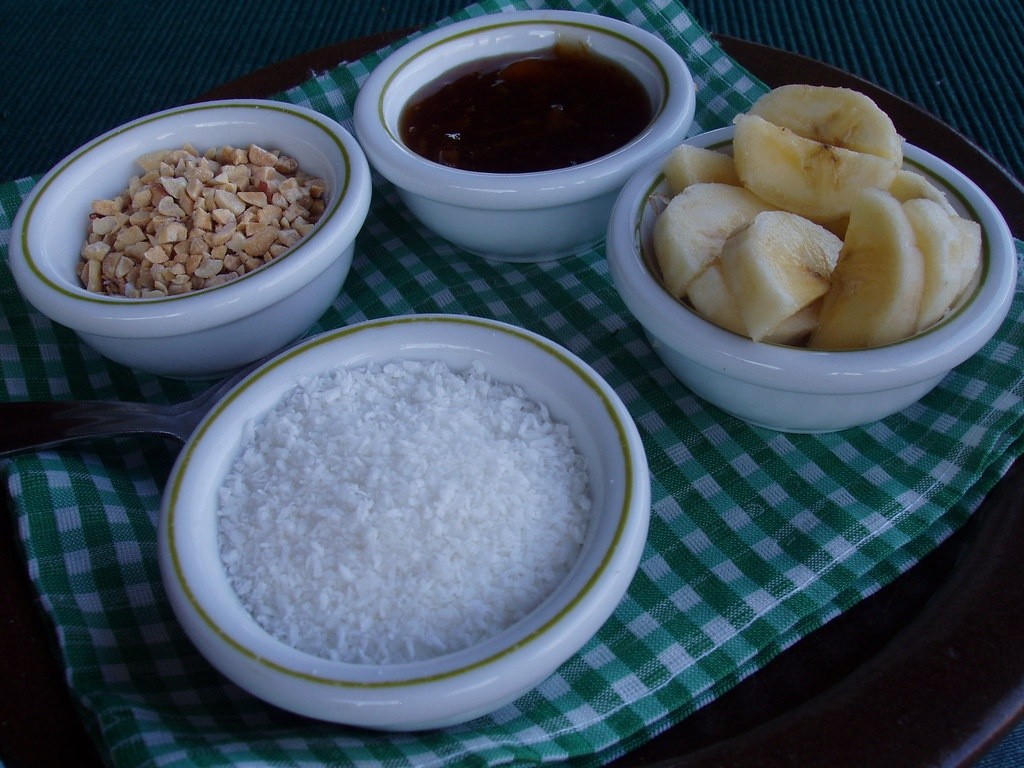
[648,83,984,350]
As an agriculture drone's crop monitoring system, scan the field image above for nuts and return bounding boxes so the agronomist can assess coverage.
[81,145,325,298]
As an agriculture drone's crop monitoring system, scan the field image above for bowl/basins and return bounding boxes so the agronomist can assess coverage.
[10,97,370,378]
[158,312,654,727]
[354,10,695,265]
[605,116,1018,434]
